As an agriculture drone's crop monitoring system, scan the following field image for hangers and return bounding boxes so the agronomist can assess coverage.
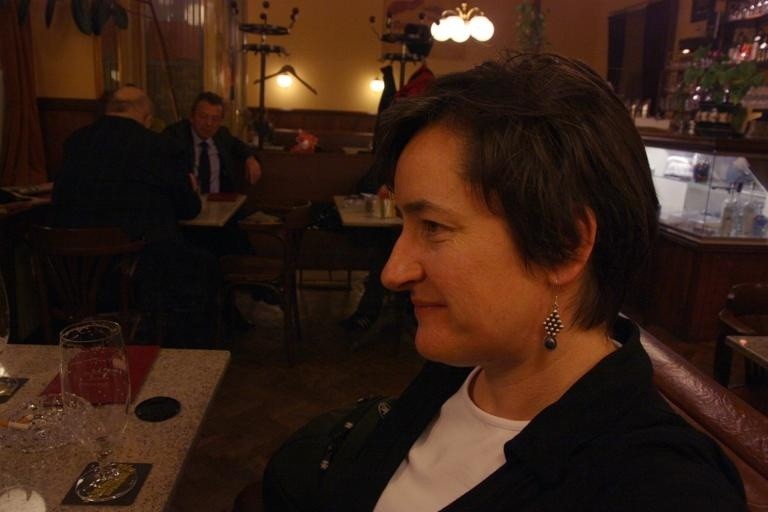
[251,55,321,97]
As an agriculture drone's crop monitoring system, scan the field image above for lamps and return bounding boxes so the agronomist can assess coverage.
[429,0,500,46]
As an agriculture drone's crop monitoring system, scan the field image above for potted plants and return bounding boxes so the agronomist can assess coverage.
[685,43,766,131]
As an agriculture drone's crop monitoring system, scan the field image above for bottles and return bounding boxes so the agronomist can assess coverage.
[623,1,768,135]
[658,153,768,237]
[237,108,276,145]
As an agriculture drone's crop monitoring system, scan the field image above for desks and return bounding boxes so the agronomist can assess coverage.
[171,189,249,232]
[329,189,418,296]
[723,333,767,396]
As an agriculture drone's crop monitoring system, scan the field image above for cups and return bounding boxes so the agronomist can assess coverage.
[199,193,212,219]
[342,184,398,220]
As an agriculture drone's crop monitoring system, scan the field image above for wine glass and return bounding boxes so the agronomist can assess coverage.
[59,321,138,505]
[0,282,19,400]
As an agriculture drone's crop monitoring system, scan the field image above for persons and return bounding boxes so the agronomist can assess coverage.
[157,90,281,327]
[52,84,201,332]
[261,50,748,511]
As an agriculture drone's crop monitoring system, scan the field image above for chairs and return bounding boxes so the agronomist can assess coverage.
[293,231,364,296]
[214,215,306,342]
[715,278,768,395]
[24,224,157,347]
[602,300,768,509]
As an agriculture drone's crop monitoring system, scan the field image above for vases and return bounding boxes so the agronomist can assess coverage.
[697,99,736,135]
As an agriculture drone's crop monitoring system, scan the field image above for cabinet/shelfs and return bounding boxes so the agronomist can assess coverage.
[633,123,768,342]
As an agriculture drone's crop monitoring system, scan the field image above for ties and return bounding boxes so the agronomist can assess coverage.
[196,141,211,193]
